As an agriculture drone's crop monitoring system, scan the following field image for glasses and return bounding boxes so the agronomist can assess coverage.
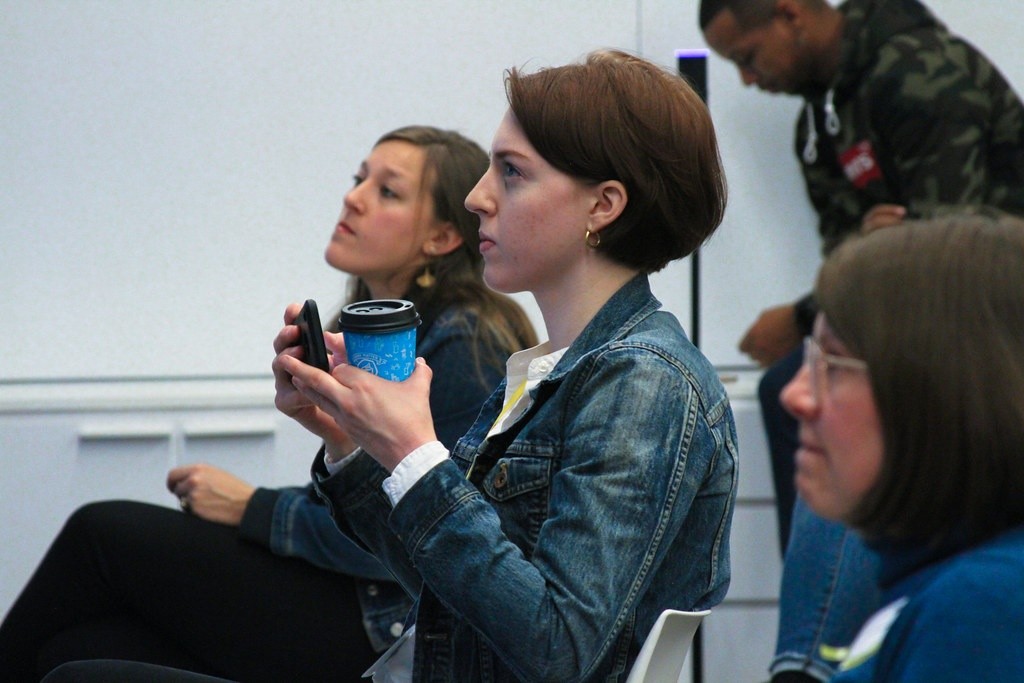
[804,337,866,387]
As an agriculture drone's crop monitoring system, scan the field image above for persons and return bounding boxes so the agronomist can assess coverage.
[695,1,1024,561]
[776,206,1024,683]
[270,46,741,682]
[0,125,541,682]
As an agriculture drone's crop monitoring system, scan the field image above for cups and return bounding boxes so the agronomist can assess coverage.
[338,299,422,382]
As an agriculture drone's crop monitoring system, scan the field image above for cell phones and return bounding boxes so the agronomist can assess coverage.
[290,299,330,379]
